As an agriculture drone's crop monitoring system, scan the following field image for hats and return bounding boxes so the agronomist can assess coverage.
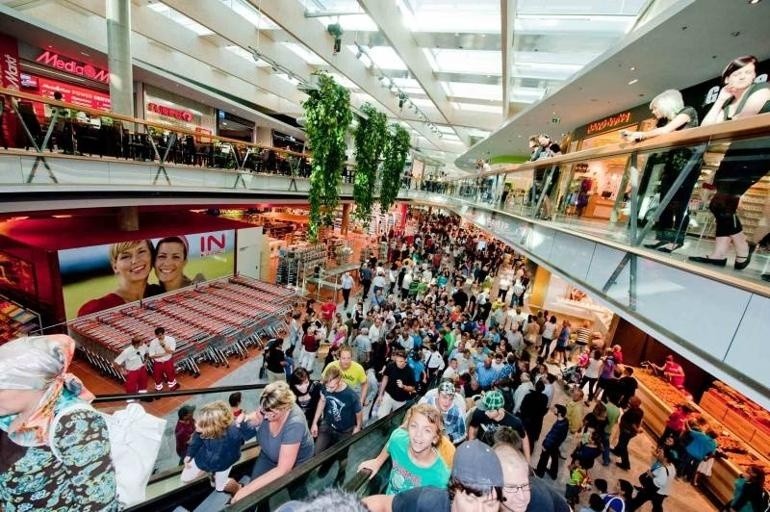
[476,391,505,410]
[452,439,505,488]
[439,382,455,395]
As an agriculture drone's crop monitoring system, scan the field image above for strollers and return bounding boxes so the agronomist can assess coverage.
[558,355,589,382]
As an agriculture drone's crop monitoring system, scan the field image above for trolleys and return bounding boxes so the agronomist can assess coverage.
[67,273,298,394]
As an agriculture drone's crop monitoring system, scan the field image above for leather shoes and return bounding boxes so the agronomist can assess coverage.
[734,240,757,270]
[689,256,727,268]
[644,240,682,253]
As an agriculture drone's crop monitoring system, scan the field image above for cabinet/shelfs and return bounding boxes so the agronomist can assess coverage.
[1,292,45,346]
[241,205,398,298]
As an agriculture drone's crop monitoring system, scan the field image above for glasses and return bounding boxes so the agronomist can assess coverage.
[505,484,532,493]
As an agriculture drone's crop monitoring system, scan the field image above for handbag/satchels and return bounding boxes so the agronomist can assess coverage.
[104,402,167,505]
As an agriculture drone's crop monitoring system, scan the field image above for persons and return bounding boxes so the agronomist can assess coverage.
[1,333,119,511]
[1,91,414,189]
[174,203,770,511]
[112,327,180,404]
[78,236,205,316]
[419,57,770,282]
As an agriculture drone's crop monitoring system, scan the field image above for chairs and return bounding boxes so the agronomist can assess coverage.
[2,95,317,179]
